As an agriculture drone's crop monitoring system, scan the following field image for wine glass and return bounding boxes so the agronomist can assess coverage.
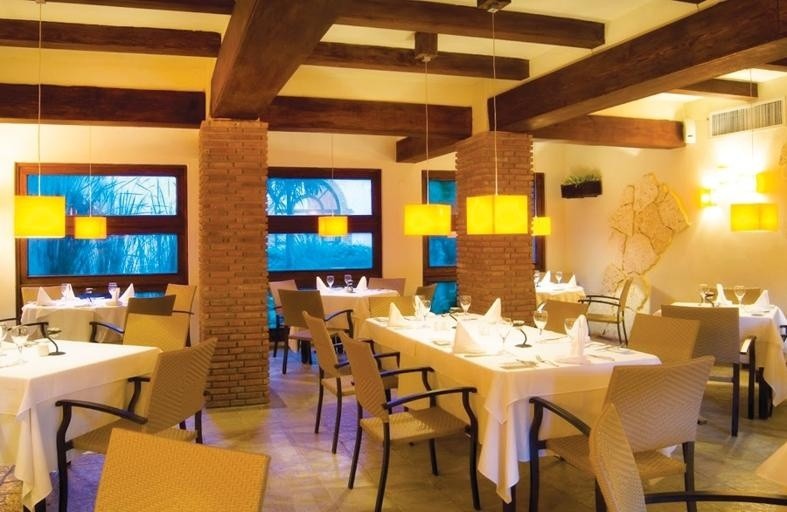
[325,274,353,294]
[533,269,563,290]
[59,282,119,306]
[1,321,31,365]
[414,294,582,361]
[696,285,745,314]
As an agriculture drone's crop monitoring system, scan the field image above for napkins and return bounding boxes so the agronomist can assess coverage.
[547,314,591,362]
[388,302,412,328]
[413,295,436,319]
[452,298,513,357]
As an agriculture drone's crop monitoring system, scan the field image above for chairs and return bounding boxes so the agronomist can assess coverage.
[0,480,22,512]
[269,274,438,375]
[338,330,481,512]
[56,336,219,512]
[588,403,787,512]
[89,283,197,351]
[95,427,271,512]
[535,269,633,347]
[626,313,700,363]
[527,356,716,512]
[302,311,400,455]
[654,283,787,437]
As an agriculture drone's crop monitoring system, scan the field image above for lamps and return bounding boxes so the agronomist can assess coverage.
[73,122,108,241]
[728,65,781,234]
[317,131,349,238]
[755,165,787,198]
[464,0,532,239]
[10,0,68,242]
[404,31,452,239]
[531,171,552,238]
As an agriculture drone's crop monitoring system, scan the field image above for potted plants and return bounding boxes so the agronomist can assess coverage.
[560,171,604,199]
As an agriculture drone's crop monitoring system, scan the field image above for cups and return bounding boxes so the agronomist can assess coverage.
[37,343,50,359]
